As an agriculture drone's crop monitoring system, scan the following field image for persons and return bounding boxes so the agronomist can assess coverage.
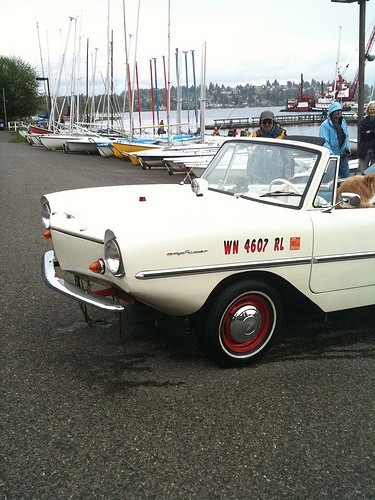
[155,120,253,138]
[320,102,352,179]
[246,110,295,185]
[356,101,375,172]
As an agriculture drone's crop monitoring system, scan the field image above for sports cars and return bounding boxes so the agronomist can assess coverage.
[40,135,375,369]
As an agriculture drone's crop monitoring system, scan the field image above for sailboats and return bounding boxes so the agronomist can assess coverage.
[17,0,375,186]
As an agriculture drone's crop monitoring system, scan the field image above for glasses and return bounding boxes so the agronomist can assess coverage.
[262,121,272,124]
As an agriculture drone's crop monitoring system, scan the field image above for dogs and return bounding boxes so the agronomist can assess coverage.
[331,172,375,209]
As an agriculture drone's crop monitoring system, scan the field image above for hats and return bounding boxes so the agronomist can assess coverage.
[260,110,275,121]
